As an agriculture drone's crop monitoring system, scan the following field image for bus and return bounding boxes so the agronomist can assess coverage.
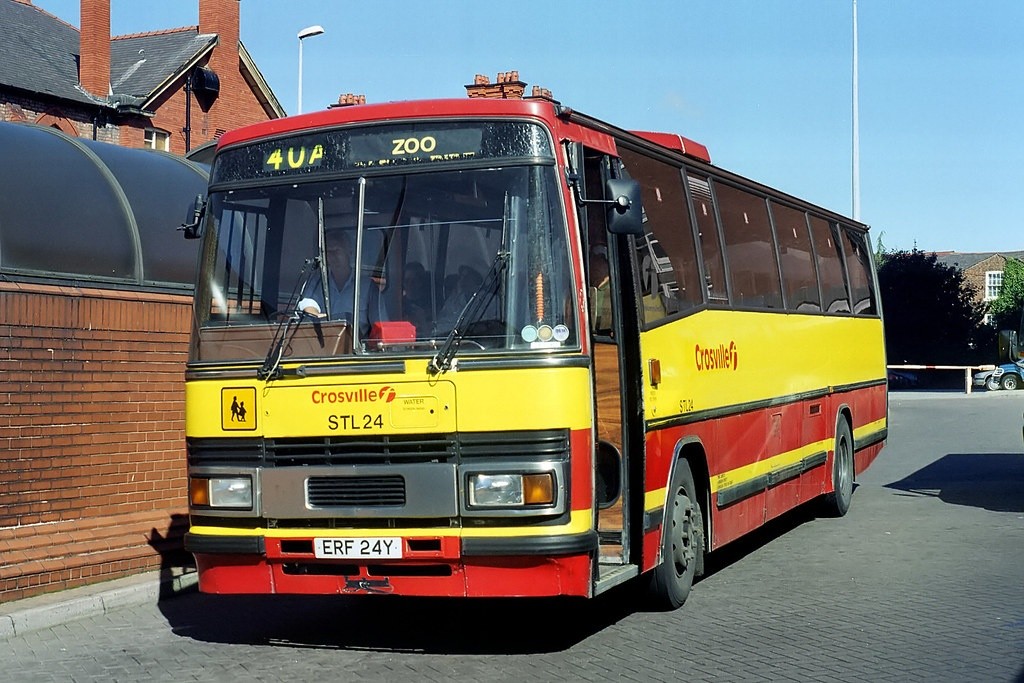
[177,71,893,614]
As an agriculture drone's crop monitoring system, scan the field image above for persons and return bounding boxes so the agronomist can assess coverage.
[369,260,500,337]
[589,252,610,288]
[297,229,390,338]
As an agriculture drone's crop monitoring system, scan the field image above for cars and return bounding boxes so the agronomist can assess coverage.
[973,359,1024,390]
[886,367,919,389]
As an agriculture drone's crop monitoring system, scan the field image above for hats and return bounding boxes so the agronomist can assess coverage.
[458,266,483,286]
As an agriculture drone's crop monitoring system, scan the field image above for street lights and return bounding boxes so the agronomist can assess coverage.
[296,25,325,116]
